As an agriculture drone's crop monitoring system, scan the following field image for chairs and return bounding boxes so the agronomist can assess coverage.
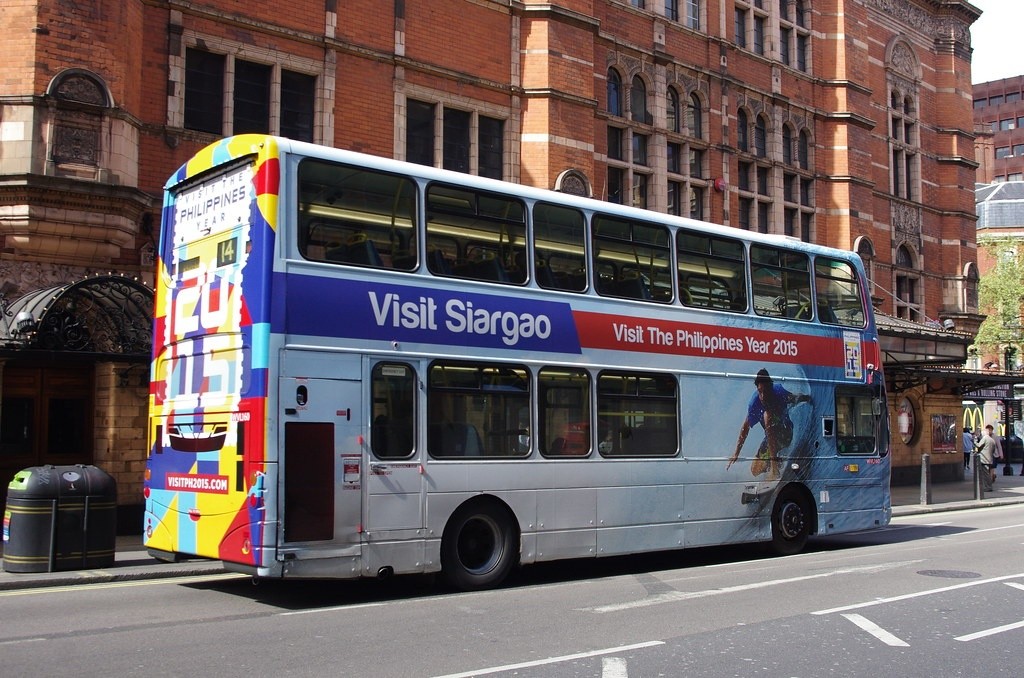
[781,303,808,319]
[346,233,385,267]
[391,249,416,269]
[453,252,694,305]
[426,243,446,272]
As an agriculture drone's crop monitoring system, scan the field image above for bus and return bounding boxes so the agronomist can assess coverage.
[144,134,891,590]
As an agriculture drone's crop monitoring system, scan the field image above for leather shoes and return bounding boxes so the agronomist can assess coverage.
[993,474,997,481]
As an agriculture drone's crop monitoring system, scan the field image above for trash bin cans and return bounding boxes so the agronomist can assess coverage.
[3,464,118,573]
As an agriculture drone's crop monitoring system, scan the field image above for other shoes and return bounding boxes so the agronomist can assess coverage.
[967,466,970,470]
[964,467,966,470]
[984,487,993,492]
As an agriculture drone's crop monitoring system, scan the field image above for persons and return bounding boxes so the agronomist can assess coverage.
[975,428,997,492]
[727,367,818,481]
[963,427,975,470]
[985,424,1006,484]
[550,409,676,457]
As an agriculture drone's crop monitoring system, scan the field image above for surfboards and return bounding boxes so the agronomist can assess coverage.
[741,470,785,504]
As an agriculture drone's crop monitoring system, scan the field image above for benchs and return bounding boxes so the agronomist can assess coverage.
[323,239,352,264]
